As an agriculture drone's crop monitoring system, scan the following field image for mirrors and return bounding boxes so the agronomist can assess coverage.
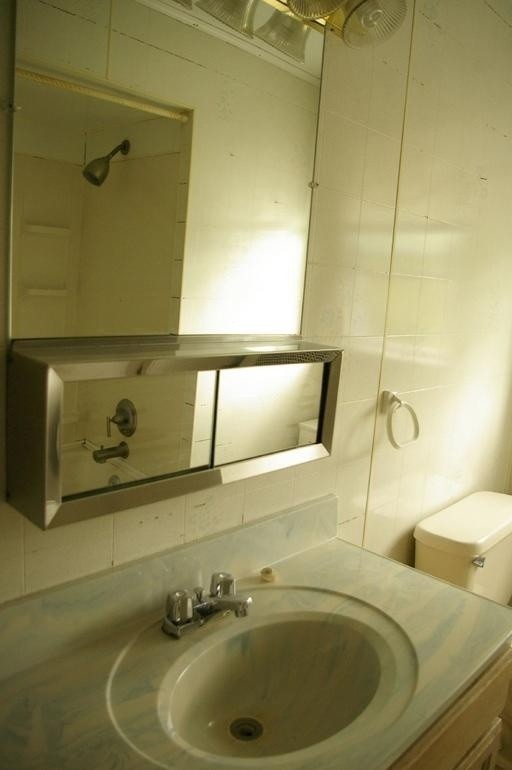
[2,1,331,336]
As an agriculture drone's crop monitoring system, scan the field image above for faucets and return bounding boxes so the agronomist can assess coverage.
[93,441,130,464]
[161,571,252,638]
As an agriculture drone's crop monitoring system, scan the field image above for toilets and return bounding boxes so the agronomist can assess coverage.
[412,492,511,605]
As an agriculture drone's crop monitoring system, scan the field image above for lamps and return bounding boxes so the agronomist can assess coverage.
[289,1,407,50]
[180,0,310,63]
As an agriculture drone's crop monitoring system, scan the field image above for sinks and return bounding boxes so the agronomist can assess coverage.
[106,587,421,770]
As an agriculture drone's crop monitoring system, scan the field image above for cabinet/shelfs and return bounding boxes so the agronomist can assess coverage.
[0,338,346,530]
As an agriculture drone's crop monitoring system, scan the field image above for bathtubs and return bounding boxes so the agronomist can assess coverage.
[57,438,149,497]
